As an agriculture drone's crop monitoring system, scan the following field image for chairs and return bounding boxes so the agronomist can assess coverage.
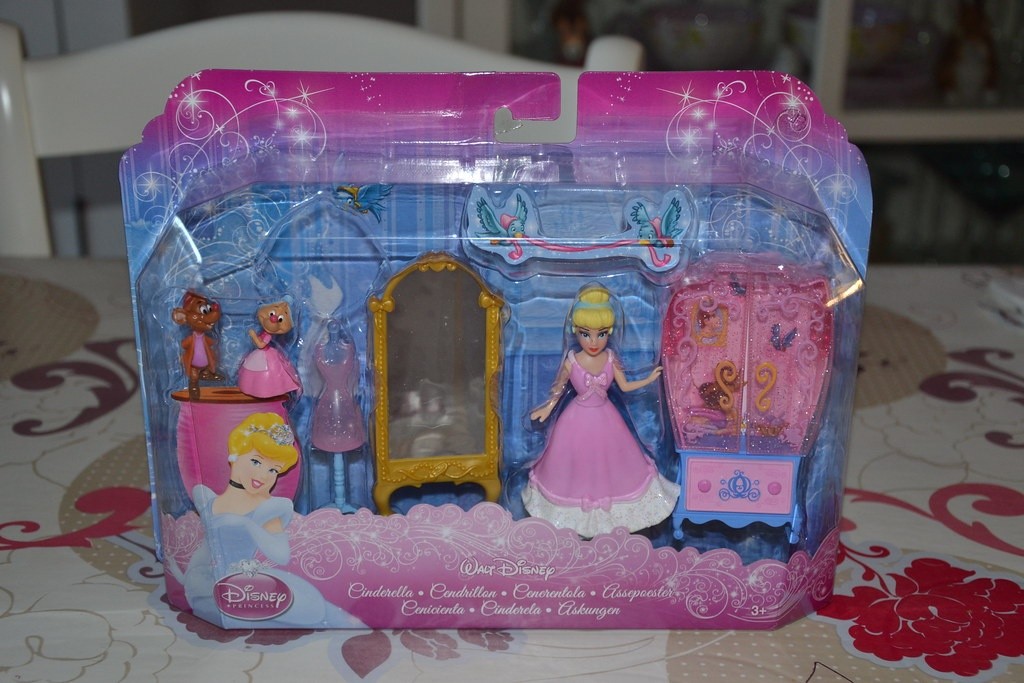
[0,13,642,260]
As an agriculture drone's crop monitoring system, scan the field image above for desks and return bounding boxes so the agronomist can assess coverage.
[0,252,1024,683]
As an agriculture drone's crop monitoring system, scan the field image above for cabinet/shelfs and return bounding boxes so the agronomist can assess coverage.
[0,0,1024,265]
